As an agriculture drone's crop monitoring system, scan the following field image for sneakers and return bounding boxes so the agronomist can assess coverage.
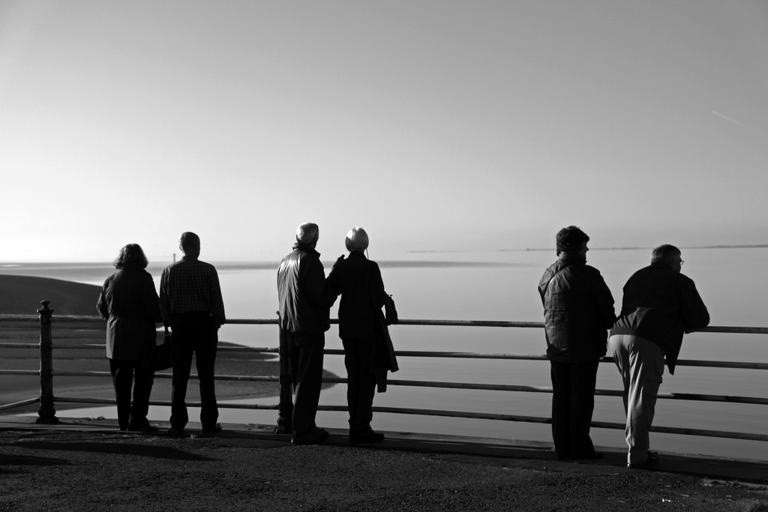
[120,419,160,431]
[350,429,385,442]
[290,427,329,445]
[204,422,222,433]
[168,427,187,438]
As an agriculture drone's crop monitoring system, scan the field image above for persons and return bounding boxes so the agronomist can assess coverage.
[534,226,615,462]
[161,232,226,431]
[606,243,712,469]
[95,244,158,429]
[276,222,332,442]
[323,227,398,441]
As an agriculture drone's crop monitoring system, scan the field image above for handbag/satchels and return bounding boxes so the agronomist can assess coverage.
[150,339,174,371]
